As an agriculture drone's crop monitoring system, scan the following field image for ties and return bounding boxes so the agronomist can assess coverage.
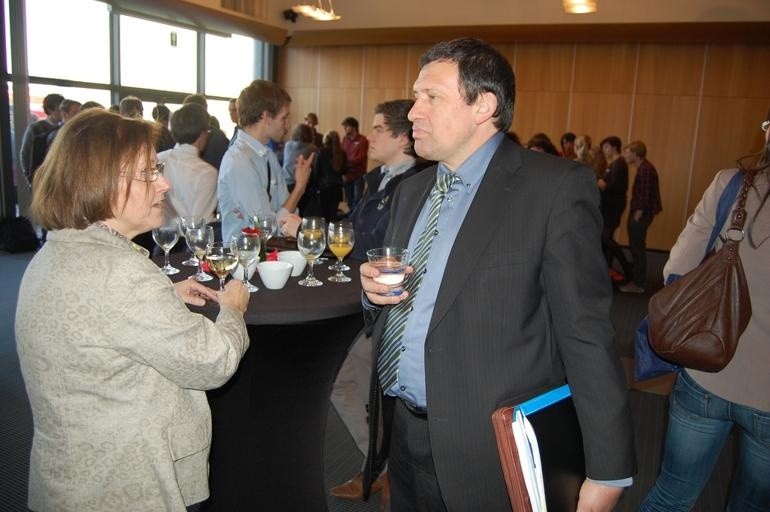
[376,173,461,395]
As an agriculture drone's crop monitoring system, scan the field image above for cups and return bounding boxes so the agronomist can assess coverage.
[225,251,308,290]
[366,247,409,298]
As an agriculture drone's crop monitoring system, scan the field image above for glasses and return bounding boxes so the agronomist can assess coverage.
[118,163,164,182]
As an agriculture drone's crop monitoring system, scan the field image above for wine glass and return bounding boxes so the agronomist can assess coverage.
[297,216,354,287]
[248,216,278,250]
[230,233,261,293]
[204,242,240,294]
[151,215,214,283]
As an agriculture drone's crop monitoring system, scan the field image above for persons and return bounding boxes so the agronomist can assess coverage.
[508,133,661,289]
[638,113,770,512]
[14,108,251,512]
[9,78,369,296]
[359,36,637,512]
[328,100,417,512]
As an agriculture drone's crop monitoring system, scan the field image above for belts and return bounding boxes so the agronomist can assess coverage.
[400,398,427,420]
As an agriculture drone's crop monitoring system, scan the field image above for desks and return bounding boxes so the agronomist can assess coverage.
[150,238,395,511]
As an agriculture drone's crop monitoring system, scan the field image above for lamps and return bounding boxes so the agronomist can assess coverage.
[292,0,340,21]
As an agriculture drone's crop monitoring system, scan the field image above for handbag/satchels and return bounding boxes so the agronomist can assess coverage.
[635,238,755,380]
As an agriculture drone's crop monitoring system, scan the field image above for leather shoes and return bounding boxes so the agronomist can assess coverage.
[620,284,645,293]
[330,472,381,499]
